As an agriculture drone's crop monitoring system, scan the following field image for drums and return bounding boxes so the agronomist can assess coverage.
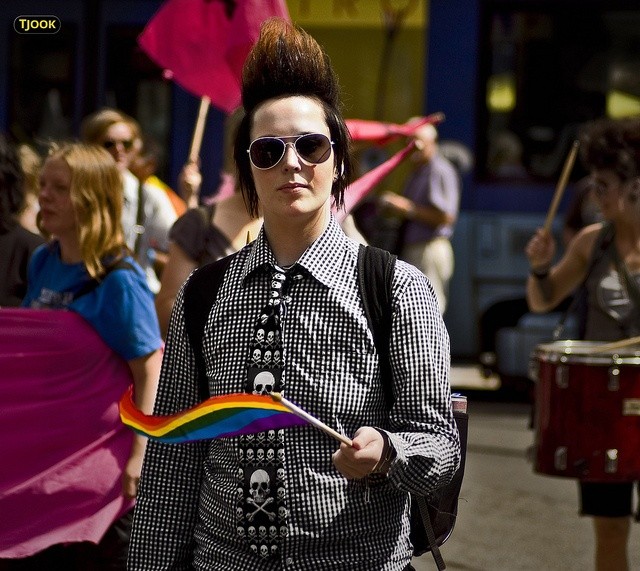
[532,338,640,482]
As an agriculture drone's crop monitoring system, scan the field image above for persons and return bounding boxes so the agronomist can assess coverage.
[563,174,596,340]
[525,117,639,571]
[380,117,460,320]
[16,144,41,236]
[129,145,188,273]
[81,109,203,295]
[154,108,264,338]
[0,142,163,571]
[127,17,462,571]
[0,142,46,309]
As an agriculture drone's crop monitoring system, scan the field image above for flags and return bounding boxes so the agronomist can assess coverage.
[120,384,309,444]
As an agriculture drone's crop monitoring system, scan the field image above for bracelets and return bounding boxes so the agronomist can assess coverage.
[531,267,549,281]
[364,438,394,503]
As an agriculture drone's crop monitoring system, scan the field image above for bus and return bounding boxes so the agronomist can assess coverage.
[422,5,639,391]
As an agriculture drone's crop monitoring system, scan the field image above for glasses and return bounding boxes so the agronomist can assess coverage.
[246,134,335,171]
[102,141,133,151]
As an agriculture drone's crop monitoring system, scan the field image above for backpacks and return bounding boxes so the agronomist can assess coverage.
[182,244,470,571]
